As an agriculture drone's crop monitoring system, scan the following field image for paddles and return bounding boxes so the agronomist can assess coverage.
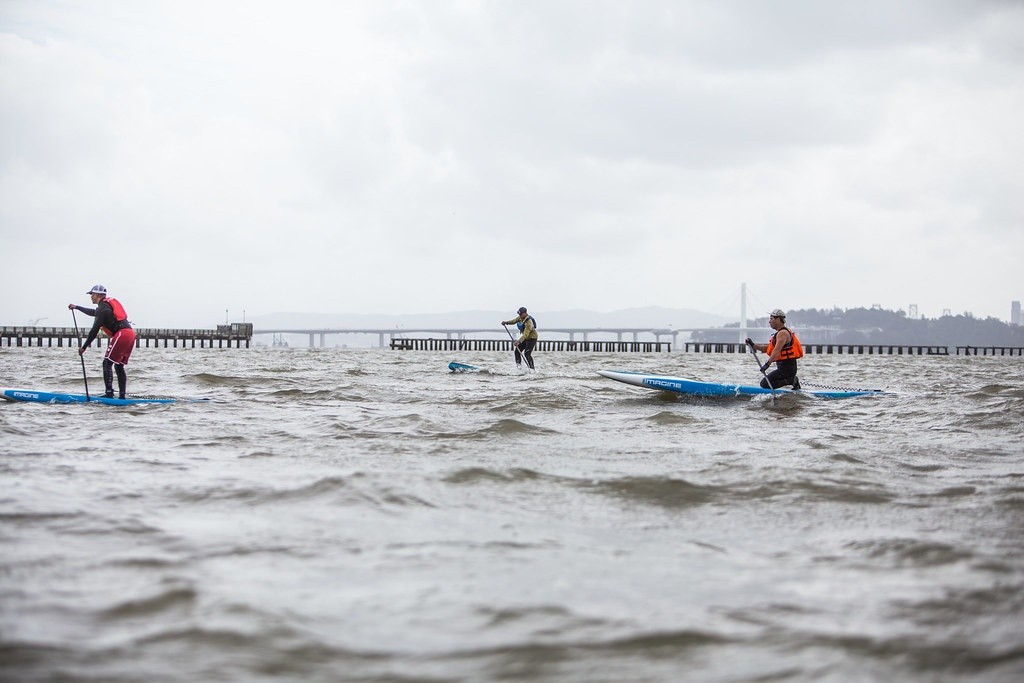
[744,339,776,395]
[69,307,92,403]
[503,324,532,373]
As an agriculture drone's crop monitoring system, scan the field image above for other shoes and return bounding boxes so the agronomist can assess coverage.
[100,393,113,398]
[119,394,125,399]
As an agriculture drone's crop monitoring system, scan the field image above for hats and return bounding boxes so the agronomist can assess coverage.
[86,285,107,294]
[767,309,786,317]
[517,307,527,315]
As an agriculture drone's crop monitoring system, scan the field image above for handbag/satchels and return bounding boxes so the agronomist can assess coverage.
[517,321,524,334]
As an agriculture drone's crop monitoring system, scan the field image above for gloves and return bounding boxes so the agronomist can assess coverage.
[760,361,771,372]
[746,338,754,348]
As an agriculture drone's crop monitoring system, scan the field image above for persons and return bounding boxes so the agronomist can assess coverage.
[747,309,803,390]
[502,307,538,370]
[69,285,135,399]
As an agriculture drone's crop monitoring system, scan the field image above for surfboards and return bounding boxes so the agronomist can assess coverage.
[595,368,894,401]
[0,386,216,408]
[448,361,501,376]
